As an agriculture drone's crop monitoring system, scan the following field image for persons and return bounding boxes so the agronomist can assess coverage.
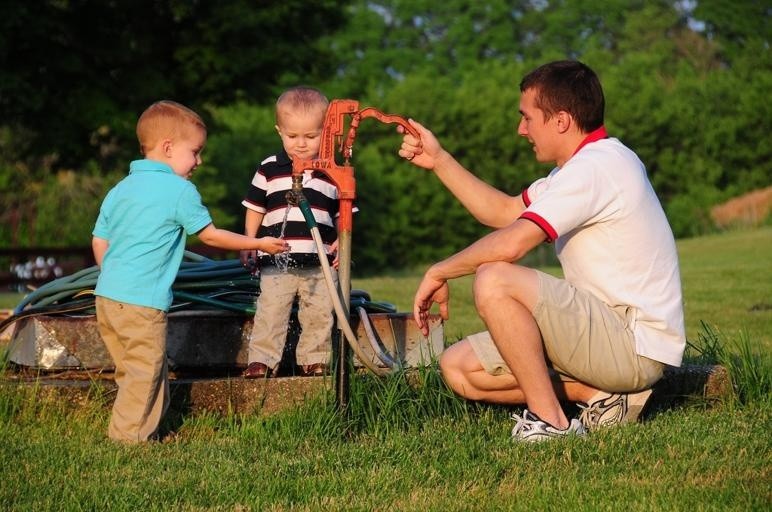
[90,97,292,447]
[239,88,361,377]
[394,59,688,444]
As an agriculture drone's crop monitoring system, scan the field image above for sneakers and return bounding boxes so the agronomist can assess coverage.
[306,363,329,376]
[245,362,271,379]
[511,388,653,443]
